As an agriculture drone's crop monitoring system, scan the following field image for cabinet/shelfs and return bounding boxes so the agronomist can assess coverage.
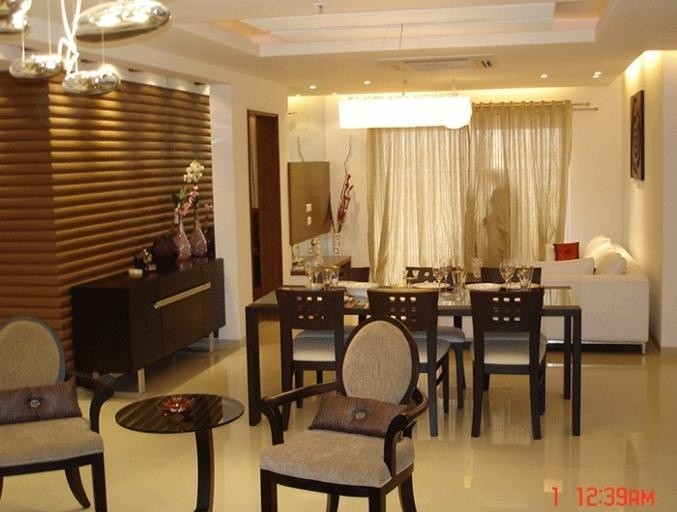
[291,255,371,283]
[69,255,226,401]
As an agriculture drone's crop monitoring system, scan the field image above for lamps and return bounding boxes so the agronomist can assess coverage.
[0,0,168,95]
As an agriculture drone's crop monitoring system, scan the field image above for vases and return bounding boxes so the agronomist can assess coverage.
[173,211,191,260]
[188,213,207,256]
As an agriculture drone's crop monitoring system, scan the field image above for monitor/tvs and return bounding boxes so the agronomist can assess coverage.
[287,162,331,244]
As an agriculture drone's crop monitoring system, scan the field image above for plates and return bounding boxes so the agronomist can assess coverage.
[464,282,499,292]
[413,281,450,290]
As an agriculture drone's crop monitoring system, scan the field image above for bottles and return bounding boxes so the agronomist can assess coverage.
[149,234,177,263]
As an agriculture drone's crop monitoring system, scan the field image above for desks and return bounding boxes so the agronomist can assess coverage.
[117,393,246,512]
[244,282,583,429]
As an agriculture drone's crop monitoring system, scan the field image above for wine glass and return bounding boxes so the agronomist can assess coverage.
[306,263,338,291]
[497,258,534,290]
[432,258,466,300]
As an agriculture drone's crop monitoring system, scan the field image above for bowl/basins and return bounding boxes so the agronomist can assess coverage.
[344,281,381,296]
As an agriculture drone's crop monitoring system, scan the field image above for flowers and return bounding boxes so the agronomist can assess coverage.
[296,136,355,255]
[169,160,217,213]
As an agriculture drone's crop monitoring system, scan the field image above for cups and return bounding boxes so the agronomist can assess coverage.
[133,255,143,270]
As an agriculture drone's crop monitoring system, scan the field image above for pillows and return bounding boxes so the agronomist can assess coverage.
[553,241,579,261]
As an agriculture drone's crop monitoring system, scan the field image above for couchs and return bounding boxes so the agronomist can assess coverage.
[508,231,649,353]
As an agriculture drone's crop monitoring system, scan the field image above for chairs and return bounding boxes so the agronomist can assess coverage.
[468,287,547,438]
[369,287,446,436]
[487,261,540,341]
[0,321,113,512]
[271,284,343,429]
[258,317,429,511]
[406,261,468,404]
